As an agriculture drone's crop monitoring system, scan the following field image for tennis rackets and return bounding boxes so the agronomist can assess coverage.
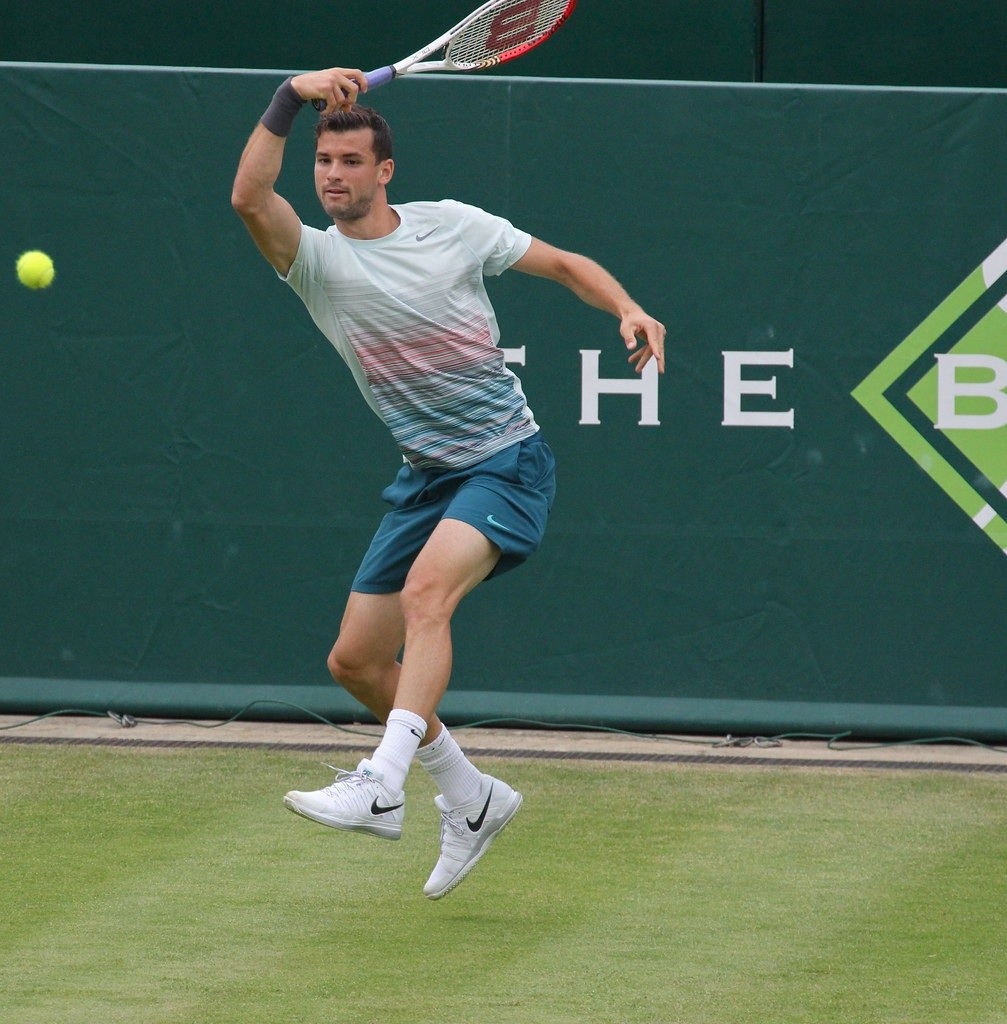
[310,0,576,113]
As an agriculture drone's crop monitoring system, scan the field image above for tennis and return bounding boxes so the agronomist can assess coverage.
[17,249,57,290]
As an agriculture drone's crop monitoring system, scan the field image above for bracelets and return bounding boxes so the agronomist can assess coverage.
[259,75,308,138]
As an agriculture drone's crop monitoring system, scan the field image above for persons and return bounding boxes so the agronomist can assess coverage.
[231,68,669,899]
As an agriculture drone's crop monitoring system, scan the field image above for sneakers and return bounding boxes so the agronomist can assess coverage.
[421,773,522,902]
[282,758,407,840]
